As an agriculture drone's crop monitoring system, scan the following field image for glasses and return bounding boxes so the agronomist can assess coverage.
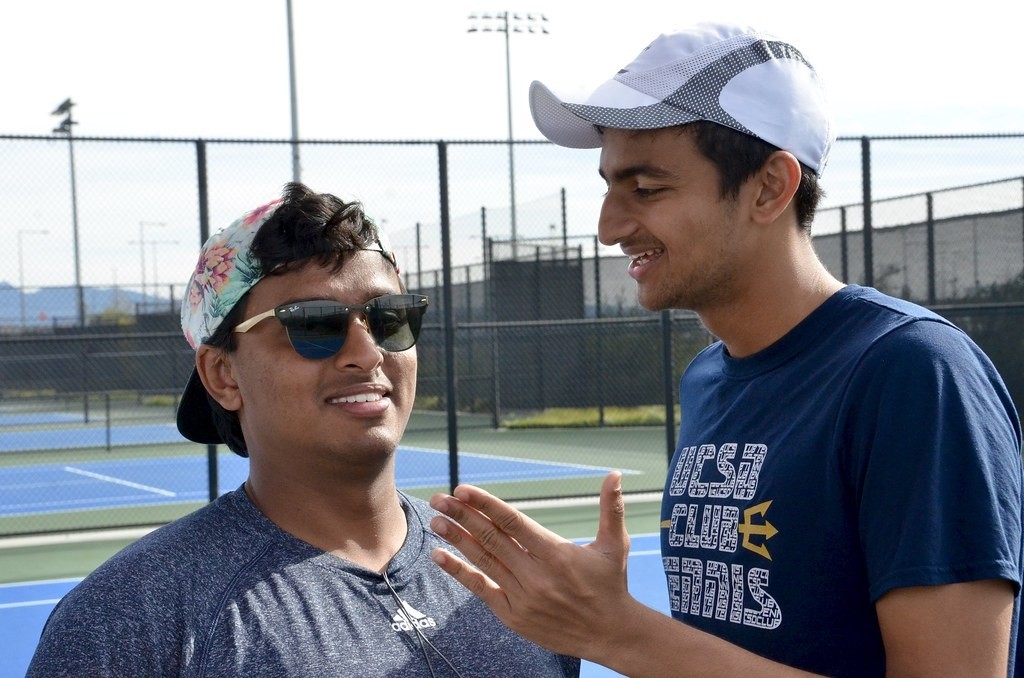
[232,295,428,358]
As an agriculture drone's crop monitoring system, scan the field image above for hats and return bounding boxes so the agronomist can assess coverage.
[176,198,400,444]
[529,19,838,179]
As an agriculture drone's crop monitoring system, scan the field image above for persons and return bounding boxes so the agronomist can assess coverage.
[25,181,584,678]
[429,22,1024,678]
